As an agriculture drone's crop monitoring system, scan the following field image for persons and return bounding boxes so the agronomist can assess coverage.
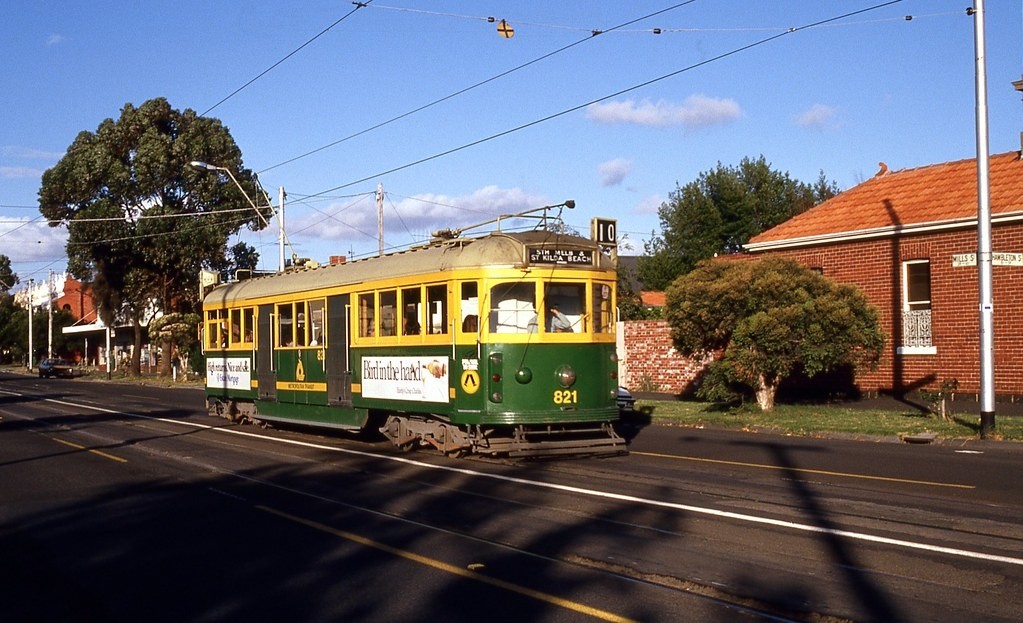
[404,312,421,335]
[528,295,571,335]
[210,320,325,350]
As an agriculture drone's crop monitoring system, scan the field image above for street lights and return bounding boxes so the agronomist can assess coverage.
[190,160,287,278]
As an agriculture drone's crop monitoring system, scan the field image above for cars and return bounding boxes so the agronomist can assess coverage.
[608,383,638,418]
[39,359,72,378]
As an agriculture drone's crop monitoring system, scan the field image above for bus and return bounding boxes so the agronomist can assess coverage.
[200,198,631,463]
